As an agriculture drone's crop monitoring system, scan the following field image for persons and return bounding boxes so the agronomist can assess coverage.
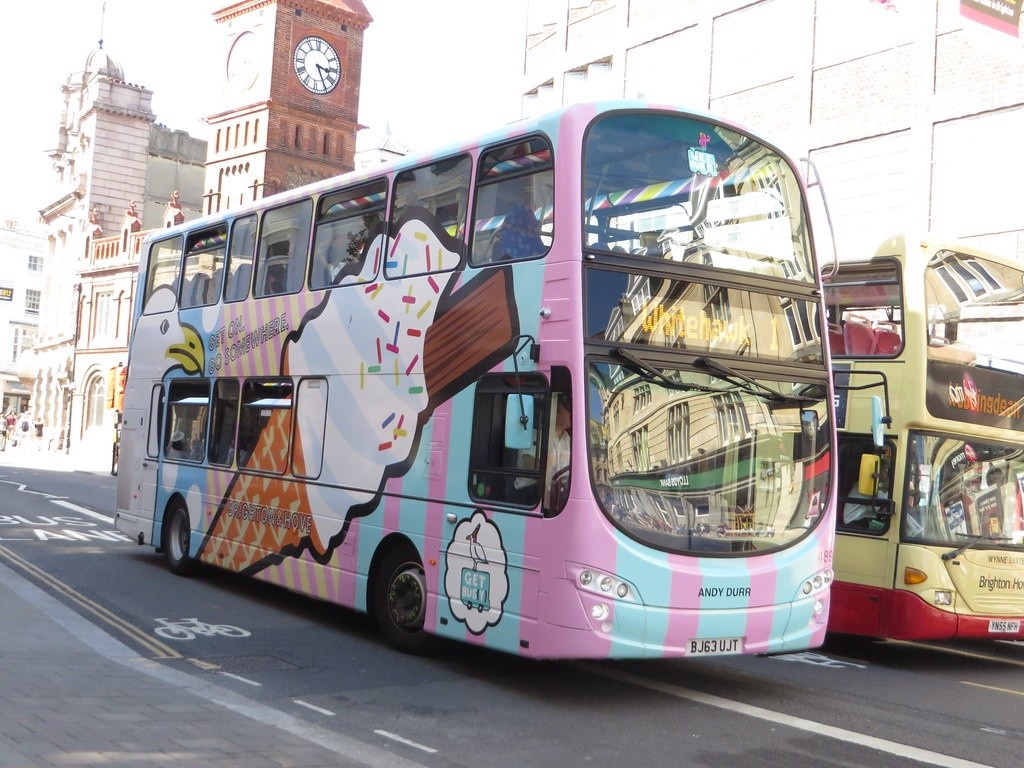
[843,459,922,530]
[977,469,1020,538]
[0,411,43,452]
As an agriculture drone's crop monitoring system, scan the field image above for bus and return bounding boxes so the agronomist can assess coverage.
[111,102,892,661]
[818,237,1023,652]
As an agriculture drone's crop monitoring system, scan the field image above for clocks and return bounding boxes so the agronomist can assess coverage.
[292,35,343,96]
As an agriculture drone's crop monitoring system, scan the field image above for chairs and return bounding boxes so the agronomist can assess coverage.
[873,320,901,355]
[828,322,845,354]
[492,203,546,262]
[171,245,340,307]
[844,312,876,355]
